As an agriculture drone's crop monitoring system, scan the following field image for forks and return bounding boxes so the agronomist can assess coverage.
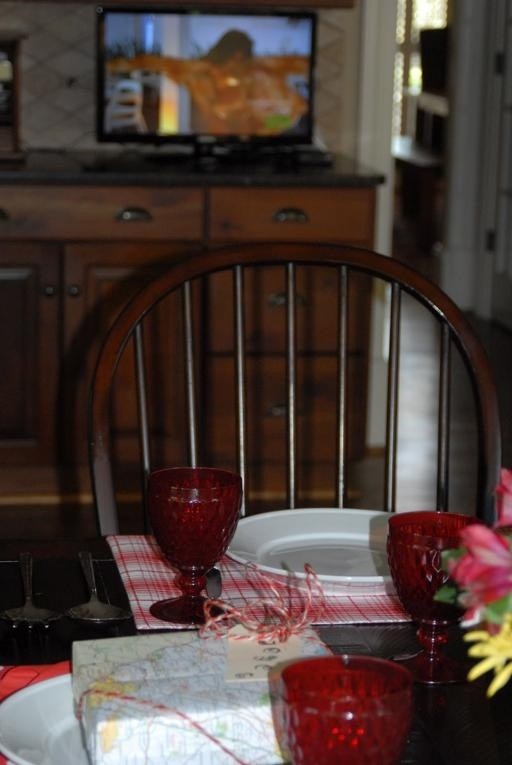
[1,551,62,630]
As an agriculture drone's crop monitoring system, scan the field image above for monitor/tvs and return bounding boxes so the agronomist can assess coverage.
[94,5,318,172]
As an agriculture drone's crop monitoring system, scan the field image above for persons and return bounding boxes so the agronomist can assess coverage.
[101,27,310,136]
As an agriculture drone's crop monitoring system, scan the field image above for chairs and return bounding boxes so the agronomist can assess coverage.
[52,238,503,563]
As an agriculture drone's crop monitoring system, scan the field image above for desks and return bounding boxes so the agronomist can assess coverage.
[1,537,511,765]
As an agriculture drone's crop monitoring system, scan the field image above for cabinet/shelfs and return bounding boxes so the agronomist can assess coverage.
[2,182,375,505]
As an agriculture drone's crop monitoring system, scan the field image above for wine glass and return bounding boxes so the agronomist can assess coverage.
[384,511,478,686]
[145,467,243,625]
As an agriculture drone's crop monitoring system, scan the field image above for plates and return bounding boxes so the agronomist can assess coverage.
[223,508,403,596]
[0,670,92,765]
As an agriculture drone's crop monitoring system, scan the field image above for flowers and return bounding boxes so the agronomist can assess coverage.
[430,467,511,700]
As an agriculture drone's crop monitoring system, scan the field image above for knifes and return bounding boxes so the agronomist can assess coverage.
[205,568,222,601]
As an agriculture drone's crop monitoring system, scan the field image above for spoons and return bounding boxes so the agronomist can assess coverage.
[67,550,134,623]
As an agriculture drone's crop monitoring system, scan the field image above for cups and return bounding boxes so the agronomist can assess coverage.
[281,655,413,765]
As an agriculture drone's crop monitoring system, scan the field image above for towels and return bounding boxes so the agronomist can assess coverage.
[103,530,416,629]
